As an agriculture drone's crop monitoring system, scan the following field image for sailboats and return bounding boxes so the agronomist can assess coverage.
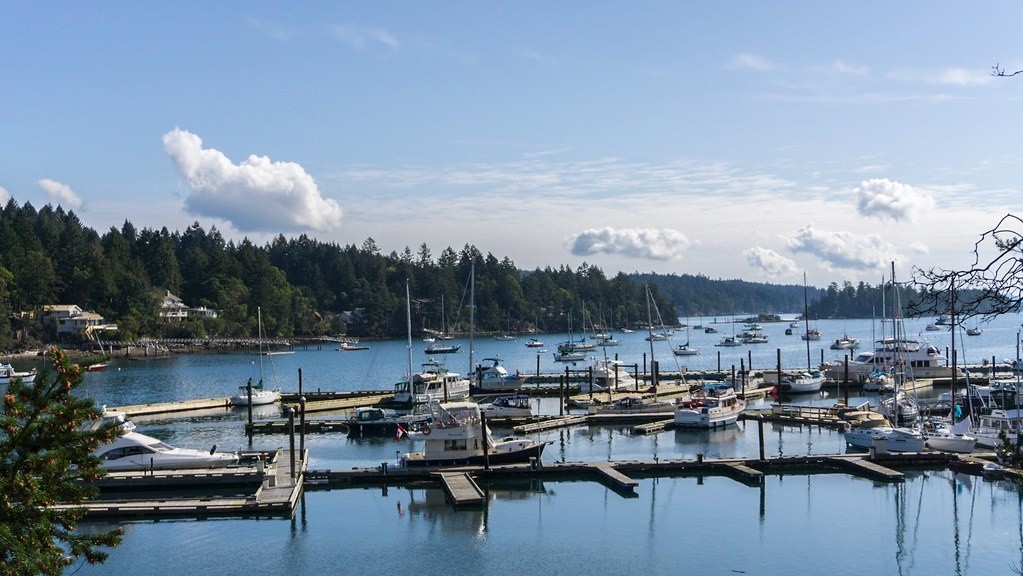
[776,271,827,393]
[437,314,455,340]
[596,283,690,413]
[966,308,984,336]
[713,301,769,347]
[346,276,471,432]
[673,315,701,356]
[494,311,515,340]
[525,315,544,347]
[822,258,1023,452]
[553,300,636,362]
[645,314,667,341]
[228,306,281,407]
[423,293,461,354]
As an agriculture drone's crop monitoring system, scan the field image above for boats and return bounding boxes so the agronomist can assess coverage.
[563,358,645,404]
[935,314,959,325]
[674,327,684,331]
[401,403,547,468]
[927,432,976,452]
[465,357,526,392]
[82,407,239,472]
[829,328,862,349]
[0,363,37,384]
[704,327,719,333]
[659,331,673,336]
[37,350,51,356]
[723,369,764,396]
[790,322,800,328]
[674,384,749,427]
[800,330,822,340]
[88,363,108,370]
[925,324,942,330]
[347,406,438,433]
[785,328,793,335]
[340,343,370,351]
[841,409,925,453]
[454,394,530,417]
[970,409,1023,448]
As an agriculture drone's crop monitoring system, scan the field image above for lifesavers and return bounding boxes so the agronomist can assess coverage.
[422,427,431,435]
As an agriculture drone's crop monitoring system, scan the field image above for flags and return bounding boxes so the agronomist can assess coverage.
[395,424,405,443]
